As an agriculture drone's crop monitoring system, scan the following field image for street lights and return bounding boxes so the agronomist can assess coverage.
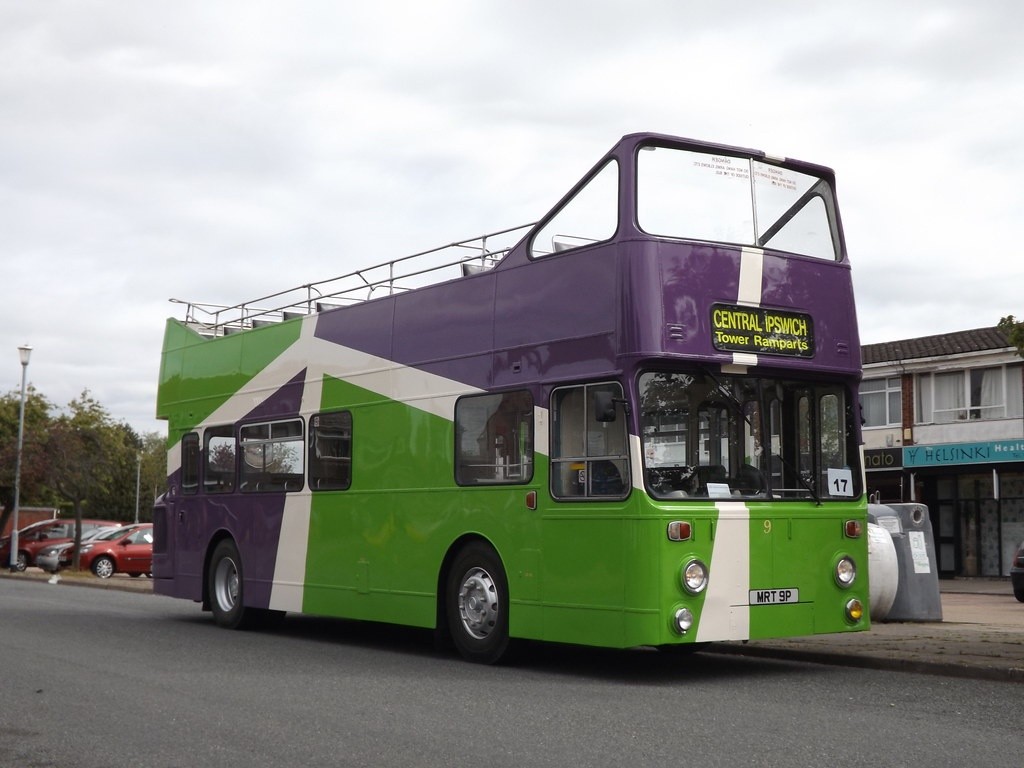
[135,452,143,524]
[8,344,35,573]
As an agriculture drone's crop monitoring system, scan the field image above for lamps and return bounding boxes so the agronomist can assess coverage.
[903,427,912,440]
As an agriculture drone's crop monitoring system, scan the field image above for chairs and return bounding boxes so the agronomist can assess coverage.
[216,459,641,500]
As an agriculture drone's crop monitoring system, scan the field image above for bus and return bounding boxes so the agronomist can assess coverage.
[153,129,871,662]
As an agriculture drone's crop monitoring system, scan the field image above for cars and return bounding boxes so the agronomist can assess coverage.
[1009,541,1024,602]
[0,519,154,579]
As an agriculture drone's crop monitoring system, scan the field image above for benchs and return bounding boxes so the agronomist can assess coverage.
[223,234,606,335]
